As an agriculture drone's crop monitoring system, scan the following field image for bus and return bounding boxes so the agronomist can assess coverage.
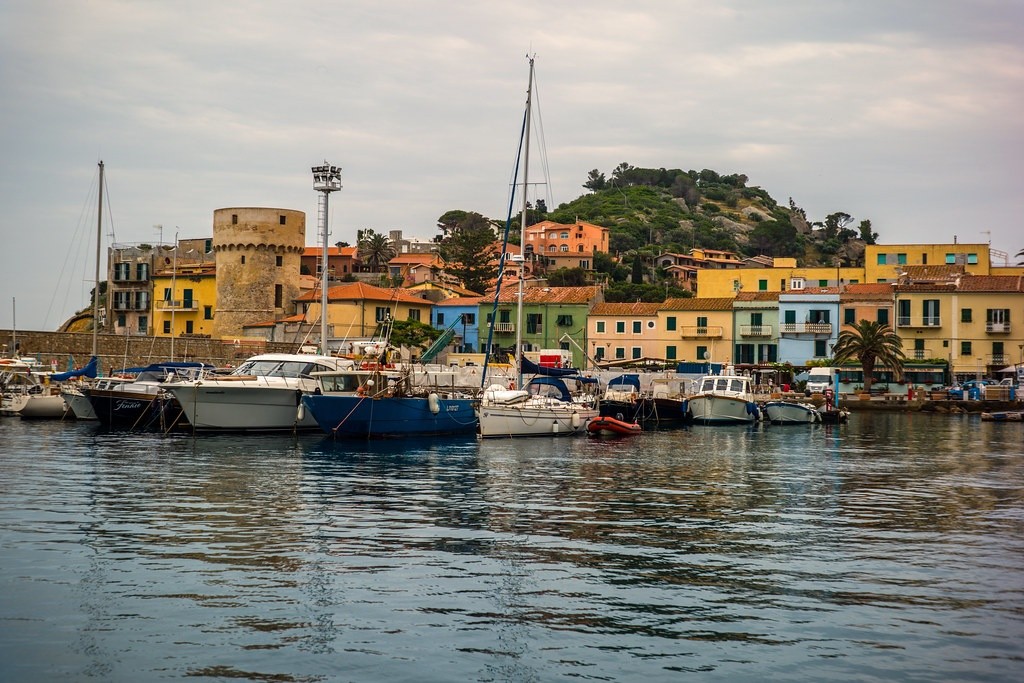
[806,363,947,395]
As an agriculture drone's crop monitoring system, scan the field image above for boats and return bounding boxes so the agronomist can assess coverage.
[0,295,851,445]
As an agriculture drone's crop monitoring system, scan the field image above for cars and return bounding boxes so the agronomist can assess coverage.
[948,381,995,400]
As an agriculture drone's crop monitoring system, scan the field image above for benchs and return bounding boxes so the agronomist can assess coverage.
[785,393,805,398]
[834,392,847,399]
[884,393,904,400]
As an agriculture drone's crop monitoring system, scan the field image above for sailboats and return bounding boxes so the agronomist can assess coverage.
[478,52,640,434]
[11,160,219,423]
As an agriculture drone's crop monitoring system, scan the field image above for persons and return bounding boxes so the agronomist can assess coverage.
[348,342,354,353]
[768,377,773,394]
[783,382,791,392]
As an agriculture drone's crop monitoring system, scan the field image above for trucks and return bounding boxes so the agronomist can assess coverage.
[804,366,836,397]
